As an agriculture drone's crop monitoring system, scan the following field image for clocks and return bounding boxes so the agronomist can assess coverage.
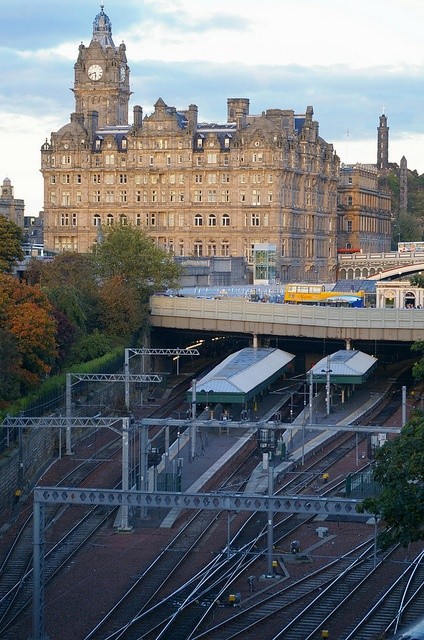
[117,64,127,83]
[87,63,105,82]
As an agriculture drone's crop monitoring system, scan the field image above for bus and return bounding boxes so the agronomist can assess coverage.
[284,284,364,306]
